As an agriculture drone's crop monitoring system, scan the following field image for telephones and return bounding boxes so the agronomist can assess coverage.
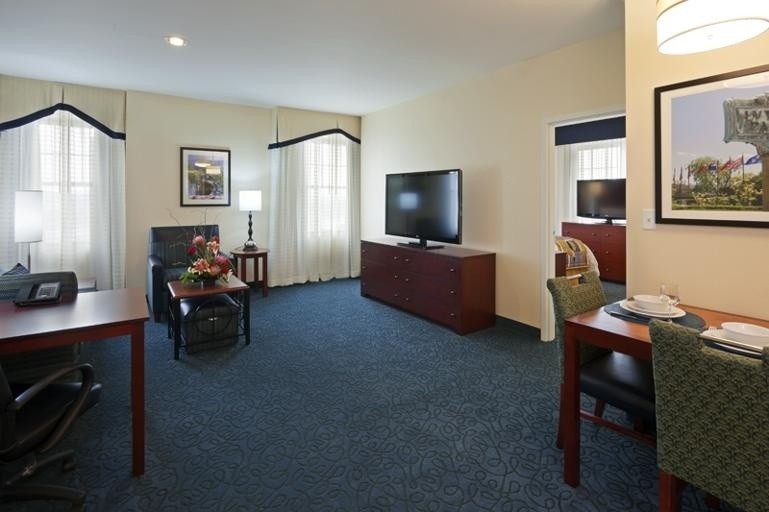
[12,281,64,306]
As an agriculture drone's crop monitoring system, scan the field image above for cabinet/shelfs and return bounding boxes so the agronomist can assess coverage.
[360,237,496,335]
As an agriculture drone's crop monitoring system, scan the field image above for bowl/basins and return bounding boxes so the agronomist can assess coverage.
[720,320,768,348]
[632,294,672,311]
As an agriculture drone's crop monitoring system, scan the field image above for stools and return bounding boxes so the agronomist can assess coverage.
[179,294,240,355]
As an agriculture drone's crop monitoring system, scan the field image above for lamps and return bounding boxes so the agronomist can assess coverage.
[13,189,45,273]
[239,190,263,252]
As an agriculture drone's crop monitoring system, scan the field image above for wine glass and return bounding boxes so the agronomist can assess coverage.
[659,283,679,324]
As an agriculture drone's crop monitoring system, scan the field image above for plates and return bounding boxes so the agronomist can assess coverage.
[619,300,686,319]
[626,302,678,315]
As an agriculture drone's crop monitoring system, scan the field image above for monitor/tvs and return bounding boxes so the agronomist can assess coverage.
[577,178,626,225]
[385,169,463,250]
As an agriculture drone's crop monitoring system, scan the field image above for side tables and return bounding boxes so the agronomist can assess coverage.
[232,246,271,298]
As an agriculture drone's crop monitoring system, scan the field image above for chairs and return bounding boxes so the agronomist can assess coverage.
[0,364,103,512]
[145,223,233,323]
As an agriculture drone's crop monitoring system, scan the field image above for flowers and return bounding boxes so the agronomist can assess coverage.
[171,225,233,287]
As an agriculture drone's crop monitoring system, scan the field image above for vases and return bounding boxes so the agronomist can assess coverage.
[201,276,216,289]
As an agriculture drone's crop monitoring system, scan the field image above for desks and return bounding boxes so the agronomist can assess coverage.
[166,276,251,359]
[0,286,151,476]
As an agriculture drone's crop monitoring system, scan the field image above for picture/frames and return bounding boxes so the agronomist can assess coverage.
[654,64,769,228]
[179,147,231,208]
